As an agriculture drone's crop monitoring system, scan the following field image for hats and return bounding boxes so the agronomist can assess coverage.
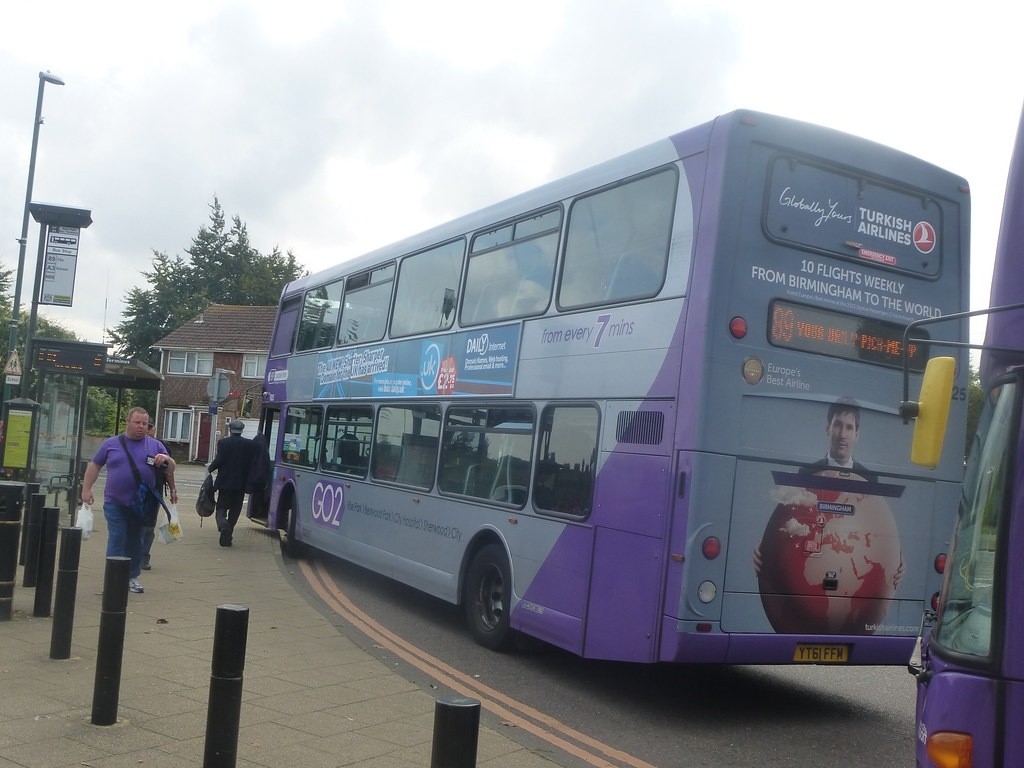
[229,421,244,433]
[148,417,154,426]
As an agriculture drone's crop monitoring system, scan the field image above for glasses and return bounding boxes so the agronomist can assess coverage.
[148,426,153,428]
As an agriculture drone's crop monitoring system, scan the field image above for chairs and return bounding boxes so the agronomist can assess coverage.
[375,442,528,505]
[602,251,665,301]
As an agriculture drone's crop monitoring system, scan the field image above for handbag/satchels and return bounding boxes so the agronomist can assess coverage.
[131,480,171,529]
[158,501,184,545]
[76,501,93,541]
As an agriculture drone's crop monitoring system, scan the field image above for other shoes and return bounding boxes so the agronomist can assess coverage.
[219,527,233,546]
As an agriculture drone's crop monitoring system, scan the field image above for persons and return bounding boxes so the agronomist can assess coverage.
[205,421,263,547]
[141,417,178,571]
[799,396,878,482]
[82,407,176,593]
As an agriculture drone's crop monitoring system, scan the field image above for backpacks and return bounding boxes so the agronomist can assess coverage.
[196,472,217,517]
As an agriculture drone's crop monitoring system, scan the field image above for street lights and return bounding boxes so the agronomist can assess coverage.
[1,67,65,473]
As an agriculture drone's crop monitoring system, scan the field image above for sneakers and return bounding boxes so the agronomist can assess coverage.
[141,554,151,569]
[128,577,144,592]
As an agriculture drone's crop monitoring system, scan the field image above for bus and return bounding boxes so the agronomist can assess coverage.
[241,107,972,665]
[898,99,1024,767]
